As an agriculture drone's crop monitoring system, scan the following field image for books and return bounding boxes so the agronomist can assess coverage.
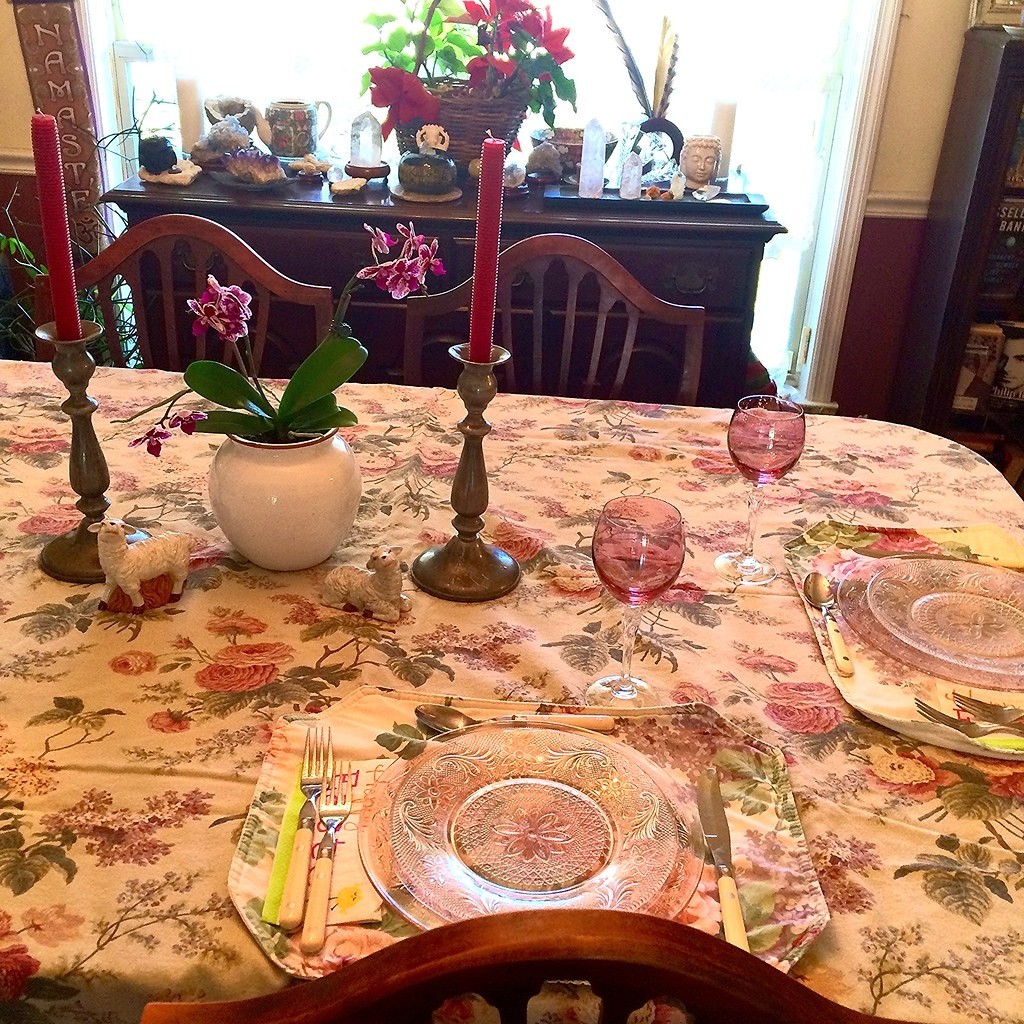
[1002,103,1024,190]
[981,196,1024,311]
[951,321,1024,487]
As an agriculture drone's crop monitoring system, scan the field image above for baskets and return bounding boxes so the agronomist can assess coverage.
[394,0,527,170]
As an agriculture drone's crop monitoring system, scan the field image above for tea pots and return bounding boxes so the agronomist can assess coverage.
[244,98,332,163]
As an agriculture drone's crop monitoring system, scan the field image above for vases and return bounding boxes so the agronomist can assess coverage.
[208,428,361,571]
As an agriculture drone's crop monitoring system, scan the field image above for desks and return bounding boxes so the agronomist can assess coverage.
[0,360,1024,1024]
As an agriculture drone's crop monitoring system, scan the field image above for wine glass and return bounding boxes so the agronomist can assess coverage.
[713,394,807,586]
[583,495,686,709]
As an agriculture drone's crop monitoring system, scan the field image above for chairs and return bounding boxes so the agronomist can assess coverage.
[34,214,333,379]
[402,230,706,406]
[143,908,925,1024]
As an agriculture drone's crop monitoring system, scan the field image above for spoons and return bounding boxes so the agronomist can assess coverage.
[415,703,617,737]
[803,571,855,678]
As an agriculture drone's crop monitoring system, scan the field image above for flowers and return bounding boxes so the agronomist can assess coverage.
[111,221,445,458]
[360,0,577,144]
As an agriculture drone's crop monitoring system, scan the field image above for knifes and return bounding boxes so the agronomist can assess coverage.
[696,767,750,954]
[853,546,1024,572]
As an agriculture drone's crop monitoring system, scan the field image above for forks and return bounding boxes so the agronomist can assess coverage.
[952,692,1024,725]
[915,698,1024,738]
[298,760,352,955]
[278,725,335,931]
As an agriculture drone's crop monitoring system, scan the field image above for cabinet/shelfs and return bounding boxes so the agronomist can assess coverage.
[887,29,1024,439]
[96,146,789,410]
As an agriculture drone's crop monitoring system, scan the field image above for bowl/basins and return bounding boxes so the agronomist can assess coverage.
[530,127,619,177]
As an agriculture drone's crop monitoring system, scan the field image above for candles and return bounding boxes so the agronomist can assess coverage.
[31,107,83,341]
[470,129,506,361]
[713,100,737,178]
[175,78,205,154]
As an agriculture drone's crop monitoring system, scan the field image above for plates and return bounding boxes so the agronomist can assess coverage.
[356,718,706,932]
[836,554,1024,693]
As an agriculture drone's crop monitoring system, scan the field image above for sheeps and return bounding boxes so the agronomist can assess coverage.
[87,517,192,615]
[320,544,413,623]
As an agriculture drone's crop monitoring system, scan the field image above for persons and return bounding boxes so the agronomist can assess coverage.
[680,131,722,189]
